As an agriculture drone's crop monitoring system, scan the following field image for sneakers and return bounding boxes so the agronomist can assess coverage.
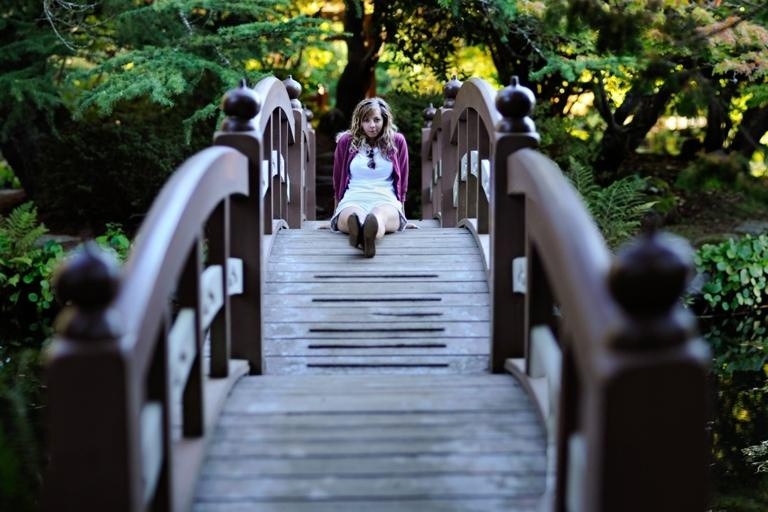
[347,212,361,247]
[361,213,377,257]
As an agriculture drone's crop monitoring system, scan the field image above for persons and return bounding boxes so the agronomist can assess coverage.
[319,97,418,257]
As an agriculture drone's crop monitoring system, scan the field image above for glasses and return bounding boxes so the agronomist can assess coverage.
[366,148,375,169]
